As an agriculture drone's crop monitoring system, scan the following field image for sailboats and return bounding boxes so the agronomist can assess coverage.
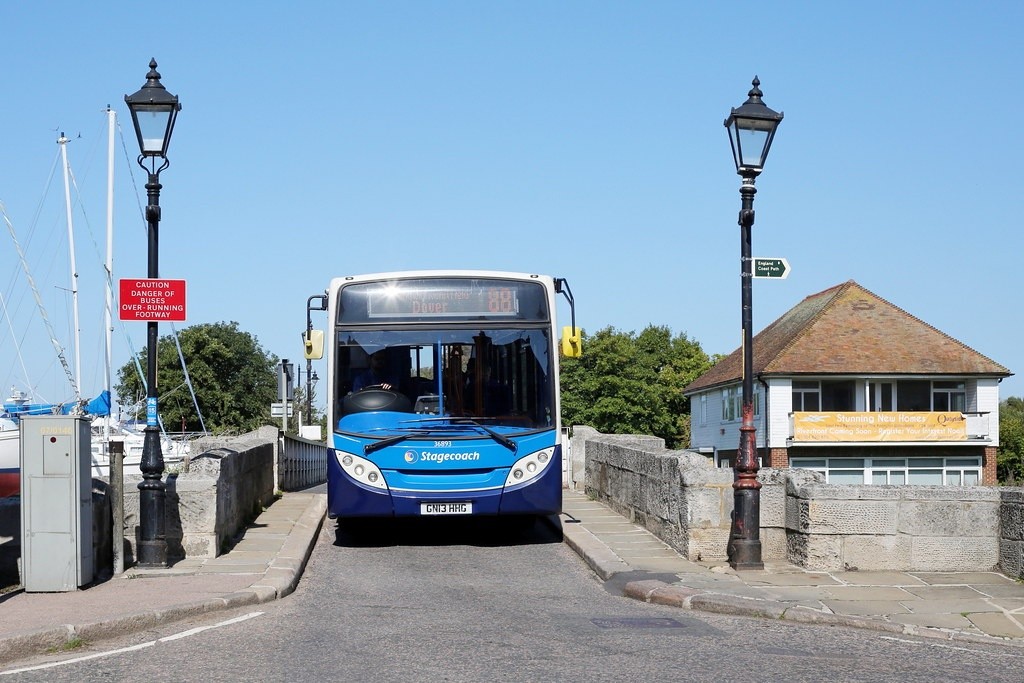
[0,107,209,545]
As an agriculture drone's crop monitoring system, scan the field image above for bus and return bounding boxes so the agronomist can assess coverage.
[300,267,585,532]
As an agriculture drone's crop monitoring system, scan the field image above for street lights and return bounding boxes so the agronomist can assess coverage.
[124,55,183,566]
[298,371,320,436]
[722,74,786,570]
